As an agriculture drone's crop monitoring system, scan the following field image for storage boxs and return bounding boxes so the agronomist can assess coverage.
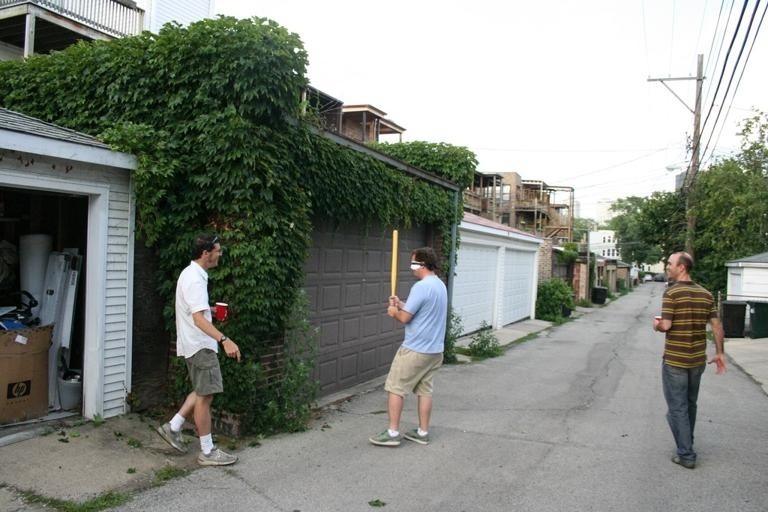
[1,324,52,424]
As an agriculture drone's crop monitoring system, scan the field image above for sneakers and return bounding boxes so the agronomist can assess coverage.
[197,446,238,466]
[156,421,190,453]
[369,428,402,446]
[403,429,430,445]
[672,454,695,469]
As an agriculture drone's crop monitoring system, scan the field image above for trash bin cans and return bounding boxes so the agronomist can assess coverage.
[722,301,747,338]
[58,369,83,410]
[592,287,608,304]
[746,300,768,339]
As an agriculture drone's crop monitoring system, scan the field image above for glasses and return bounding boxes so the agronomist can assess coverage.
[207,235,221,251]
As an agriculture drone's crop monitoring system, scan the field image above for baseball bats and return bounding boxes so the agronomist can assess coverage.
[391,230,398,297]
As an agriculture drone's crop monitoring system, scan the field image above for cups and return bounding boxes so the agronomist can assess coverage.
[215,301,229,322]
[654,315,664,323]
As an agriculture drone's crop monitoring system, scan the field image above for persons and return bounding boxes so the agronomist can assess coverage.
[653,251,728,468]
[157,235,241,466]
[368,248,448,446]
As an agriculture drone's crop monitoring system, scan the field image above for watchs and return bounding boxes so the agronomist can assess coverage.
[220,335,228,344]
